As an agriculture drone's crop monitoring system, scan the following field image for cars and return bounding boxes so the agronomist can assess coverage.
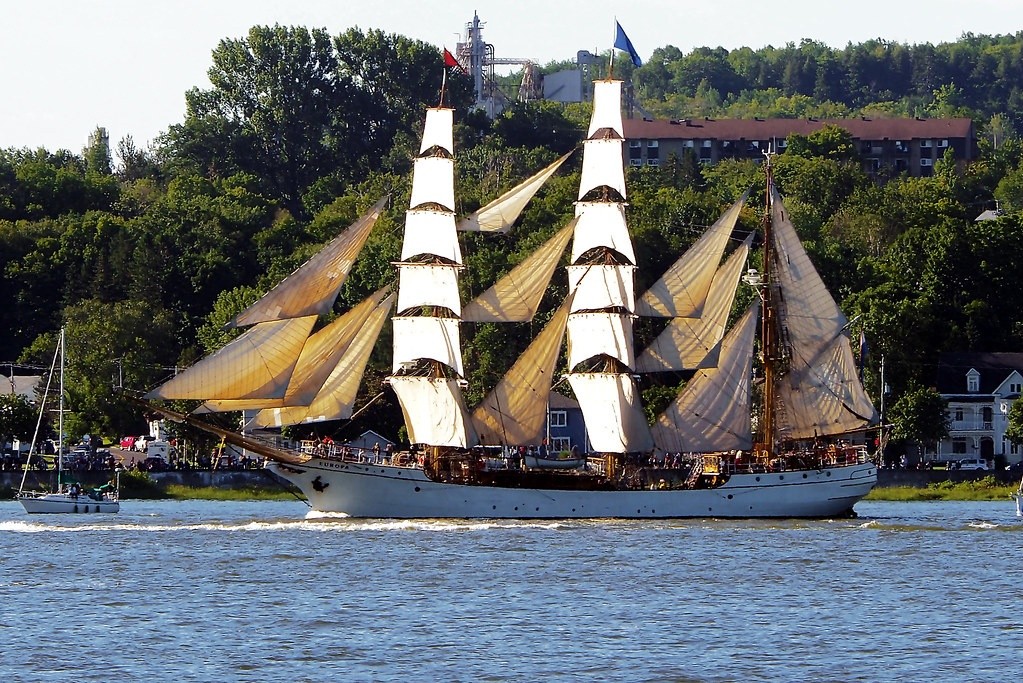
[49,431,178,470]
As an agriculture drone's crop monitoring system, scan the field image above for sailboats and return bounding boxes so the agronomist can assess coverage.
[11,326,117,514]
[120,37,892,520]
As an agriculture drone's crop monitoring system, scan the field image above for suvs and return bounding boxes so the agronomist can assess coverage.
[948,457,990,472]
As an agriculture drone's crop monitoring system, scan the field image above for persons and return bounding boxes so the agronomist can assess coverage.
[304,431,857,491]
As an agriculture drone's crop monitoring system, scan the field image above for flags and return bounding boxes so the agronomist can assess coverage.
[445,48,467,73]
[860,331,868,383]
[614,20,641,67]
